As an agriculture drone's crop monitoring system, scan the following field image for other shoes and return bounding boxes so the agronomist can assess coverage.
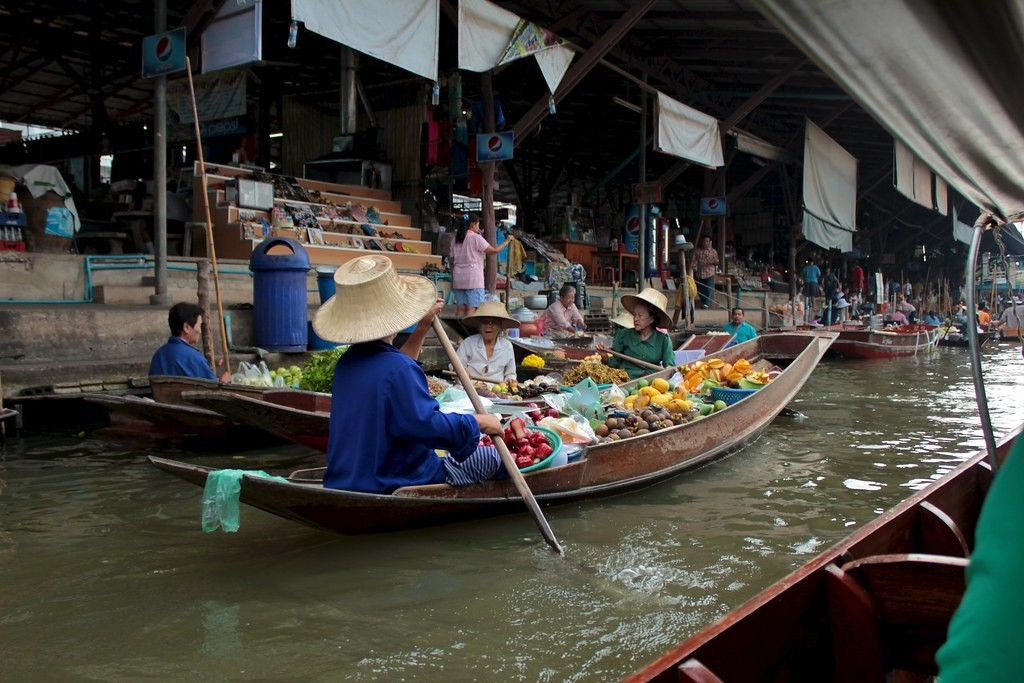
[703,304,709,309]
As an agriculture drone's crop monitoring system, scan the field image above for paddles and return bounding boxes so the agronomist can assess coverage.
[597,346,804,418]
[991,323,1001,330]
[431,312,567,555]
[440,369,509,384]
[939,320,954,345]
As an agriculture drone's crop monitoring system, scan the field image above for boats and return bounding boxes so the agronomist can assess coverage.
[130,373,266,421]
[940,332,993,348]
[178,331,737,455]
[623,427,1024,683]
[149,329,839,530]
[830,324,939,355]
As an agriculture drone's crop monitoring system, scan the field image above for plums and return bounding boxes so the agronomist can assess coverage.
[509,382,560,398]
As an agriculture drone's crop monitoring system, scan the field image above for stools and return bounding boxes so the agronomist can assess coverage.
[562,281,587,311]
[537,290,559,308]
[182,221,214,257]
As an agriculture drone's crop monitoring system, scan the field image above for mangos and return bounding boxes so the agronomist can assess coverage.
[611,378,727,423]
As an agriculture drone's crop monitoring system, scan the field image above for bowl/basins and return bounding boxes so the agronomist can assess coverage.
[522,295,548,310]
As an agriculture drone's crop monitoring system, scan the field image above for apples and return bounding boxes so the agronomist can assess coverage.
[269,365,302,385]
[492,384,509,393]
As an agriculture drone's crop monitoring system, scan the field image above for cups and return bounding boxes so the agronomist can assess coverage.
[8,193,20,213]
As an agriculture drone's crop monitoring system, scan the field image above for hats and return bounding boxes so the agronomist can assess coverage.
[462,301,521,331]
[675,234,688,244]
[621,288,672,329]
[836,298,851,309]
[311,254,440,345]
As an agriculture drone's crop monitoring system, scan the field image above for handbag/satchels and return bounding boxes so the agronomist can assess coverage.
[237,361,262,376]
[564,377,606,423]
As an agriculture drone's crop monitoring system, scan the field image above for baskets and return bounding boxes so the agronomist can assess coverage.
[710,387,761,406]
[516,425,563,473]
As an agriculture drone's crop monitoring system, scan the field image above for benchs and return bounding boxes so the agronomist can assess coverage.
[166,232,182,255]
[78,233,130,254]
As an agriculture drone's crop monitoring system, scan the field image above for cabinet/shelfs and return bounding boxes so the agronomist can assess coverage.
[545,239,598,280]
[190,160,444,274]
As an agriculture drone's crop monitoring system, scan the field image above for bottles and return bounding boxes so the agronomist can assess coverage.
[0,226,22,241]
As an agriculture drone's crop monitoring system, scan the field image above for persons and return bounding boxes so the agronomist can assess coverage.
[721,307,758,348]
[594,288,676,380]
[312,255,505,495]
[448,213,515,315]
[448,302,517,389]
[147,302,231,396]
[540,285,587,333]
[802,258,1024,330]
[690,235,720,309]
[132,149,255,257]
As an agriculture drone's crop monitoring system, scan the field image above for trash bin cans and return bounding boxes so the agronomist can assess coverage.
[248,236,313,353]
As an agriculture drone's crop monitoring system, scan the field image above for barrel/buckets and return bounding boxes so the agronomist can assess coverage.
[675,234,687,244]
[316,277,336,304]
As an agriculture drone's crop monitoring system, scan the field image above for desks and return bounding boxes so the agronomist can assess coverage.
[589,250,639,287]
[115,210,154,255]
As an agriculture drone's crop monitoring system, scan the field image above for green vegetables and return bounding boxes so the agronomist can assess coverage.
[299,346,349,392]
